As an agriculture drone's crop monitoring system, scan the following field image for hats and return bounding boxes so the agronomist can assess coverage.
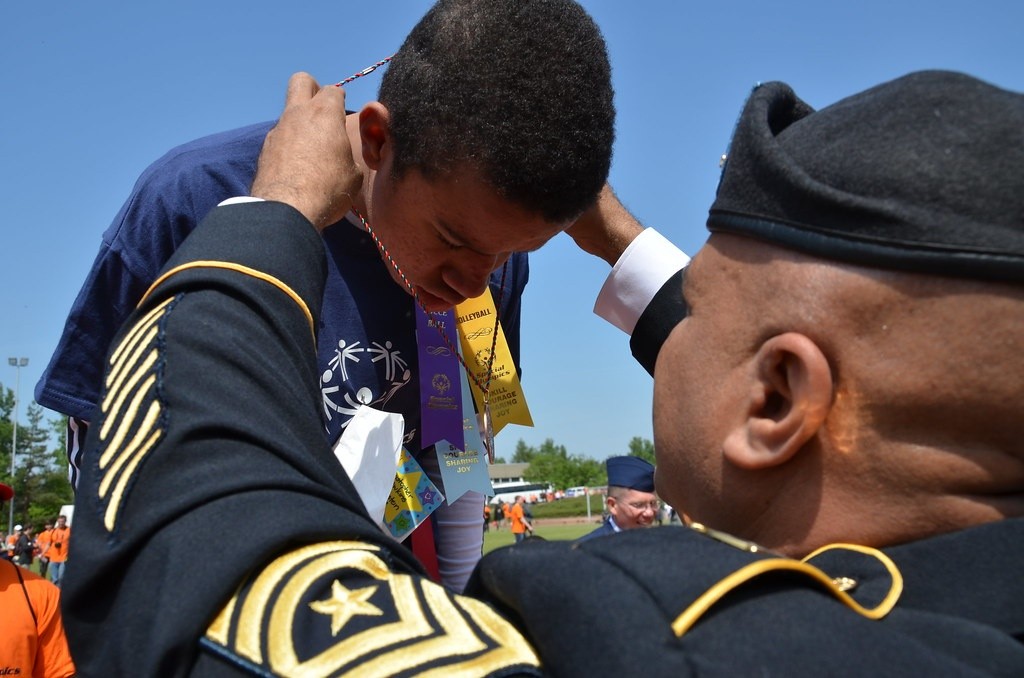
[14,524,23,530]
[0,483,14,500]
[707,69,1024,283]
[607,457,655,493]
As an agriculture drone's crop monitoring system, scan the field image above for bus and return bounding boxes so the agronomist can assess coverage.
[487,481,555,504]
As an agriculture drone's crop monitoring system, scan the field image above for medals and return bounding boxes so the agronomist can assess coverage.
[483,403,496,465]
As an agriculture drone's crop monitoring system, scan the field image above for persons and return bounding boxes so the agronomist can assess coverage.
[57,64,1024,678]
[33,1,613,591]
[0,512,72,588]
[480,455,696,546]
[0,483,77,678]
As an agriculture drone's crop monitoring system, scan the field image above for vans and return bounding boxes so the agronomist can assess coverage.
[564,486,585,499]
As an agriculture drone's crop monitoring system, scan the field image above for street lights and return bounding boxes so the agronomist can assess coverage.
[7,356,29,537]
